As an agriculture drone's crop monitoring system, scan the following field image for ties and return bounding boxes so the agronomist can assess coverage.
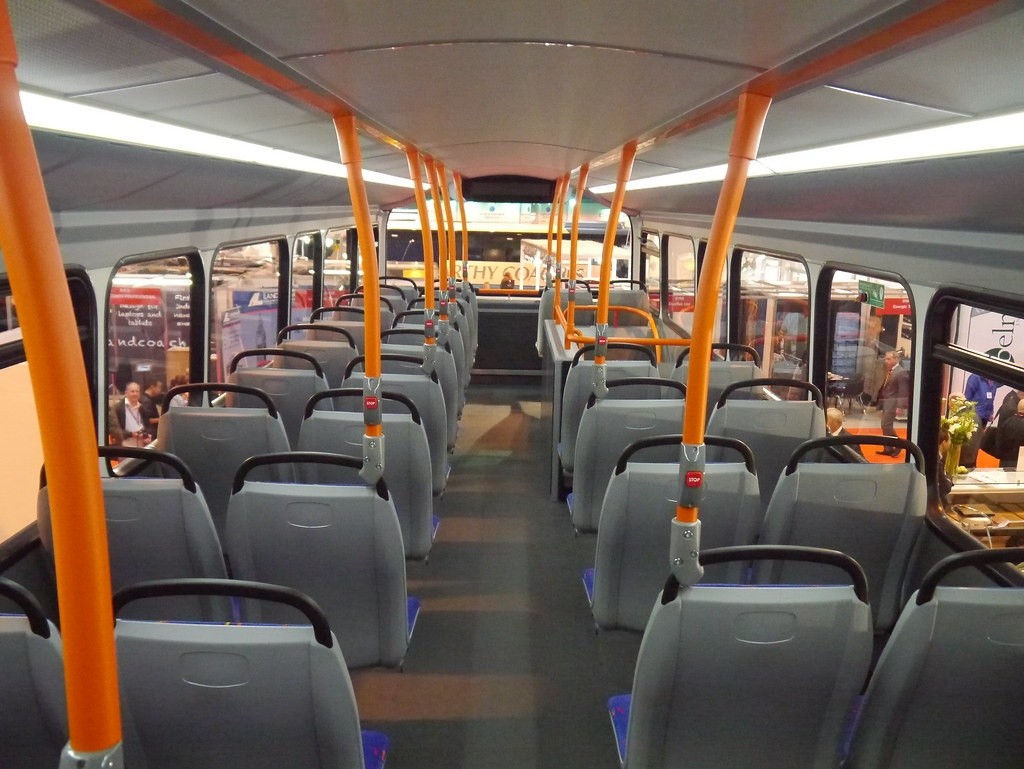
[884,369,893,388]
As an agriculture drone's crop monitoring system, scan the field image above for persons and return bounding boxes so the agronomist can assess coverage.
[959,373,1004,468]
[827,407,864,456]
[787,350,807,401]
[109,382,152,464]
[483,281,489,289]
[170,375,188,407]
[500,272,514,289]
[774,327,784,356]
[139,378,163,441]
[875,350,910,457]
[938,428,955,509]
[996,388,1024,468]
[760,316,783,356]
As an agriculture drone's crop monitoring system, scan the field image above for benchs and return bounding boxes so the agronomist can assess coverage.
[535,278,1024,769]
[0,277,477,769]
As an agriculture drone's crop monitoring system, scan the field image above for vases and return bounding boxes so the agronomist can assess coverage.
[944,439,963,482]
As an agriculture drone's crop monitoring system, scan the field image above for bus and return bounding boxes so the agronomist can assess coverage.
[384,216,632,292]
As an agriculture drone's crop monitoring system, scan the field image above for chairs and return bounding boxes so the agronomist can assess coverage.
[832,373,868,419]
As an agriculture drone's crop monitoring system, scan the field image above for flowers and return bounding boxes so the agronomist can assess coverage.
[941,396,979,467]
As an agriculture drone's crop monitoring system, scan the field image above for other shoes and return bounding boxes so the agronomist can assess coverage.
[876,451,892,456]
[892,447,902,458]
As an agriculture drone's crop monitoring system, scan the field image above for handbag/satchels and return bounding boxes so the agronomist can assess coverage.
[980,426,1003,459]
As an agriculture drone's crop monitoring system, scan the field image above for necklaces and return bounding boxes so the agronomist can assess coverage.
[127,405,139,426]
